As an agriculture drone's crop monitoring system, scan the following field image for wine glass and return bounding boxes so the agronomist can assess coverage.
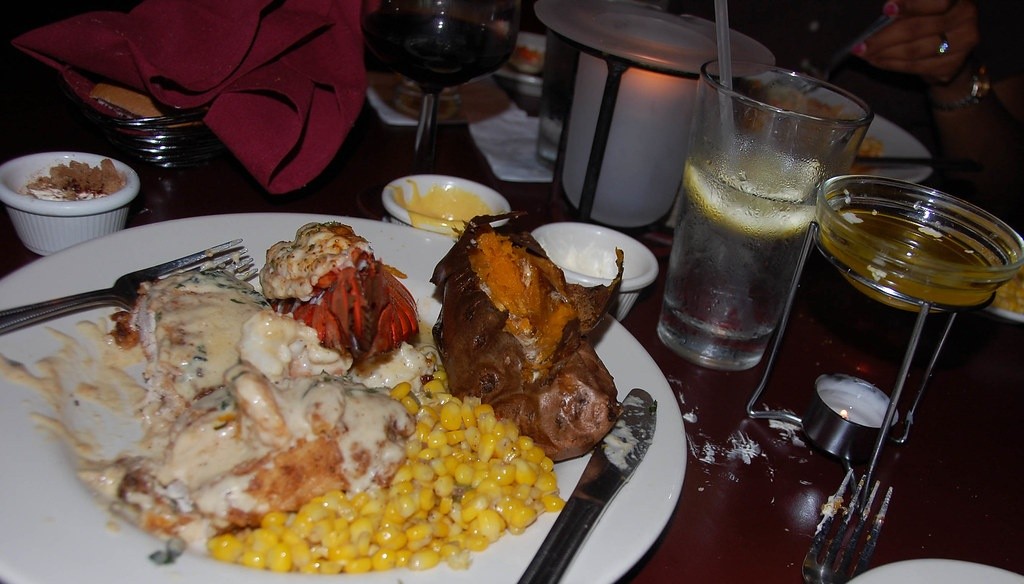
[333,0,520,202]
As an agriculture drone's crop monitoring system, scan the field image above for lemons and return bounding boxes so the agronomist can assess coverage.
[685,162,820,238]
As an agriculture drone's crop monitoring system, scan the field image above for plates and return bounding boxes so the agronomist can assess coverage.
[983,307,1024,325]
[846,559,1023,584]
[366,74,526,126]
[750,72,935,184]
[494,32,548,96]
[0,216,686,584]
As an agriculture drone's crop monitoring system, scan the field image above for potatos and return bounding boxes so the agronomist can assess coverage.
[429,208,624,460]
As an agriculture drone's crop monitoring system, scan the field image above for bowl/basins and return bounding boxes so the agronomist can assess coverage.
[530,222,659,322]
[816,175,1024,311]
[381,174,511,233]
[0,152,140,255]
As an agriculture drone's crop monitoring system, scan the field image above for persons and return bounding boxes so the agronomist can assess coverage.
[669,0,1023,207]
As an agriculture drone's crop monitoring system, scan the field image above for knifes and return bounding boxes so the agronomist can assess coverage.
[517,389,656,584]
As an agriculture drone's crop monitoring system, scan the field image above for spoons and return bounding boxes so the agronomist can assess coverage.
[788,14,889,81]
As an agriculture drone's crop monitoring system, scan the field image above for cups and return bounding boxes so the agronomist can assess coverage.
[537,0,667,172]
[656,58,873,371]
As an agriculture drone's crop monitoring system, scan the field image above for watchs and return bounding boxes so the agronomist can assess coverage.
[915,65,994,112]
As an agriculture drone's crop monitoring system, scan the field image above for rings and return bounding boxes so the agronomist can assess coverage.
[937,31,950,56]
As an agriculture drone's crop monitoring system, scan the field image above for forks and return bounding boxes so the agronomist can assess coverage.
[725,469,893,584]
[0,239,258,334]
